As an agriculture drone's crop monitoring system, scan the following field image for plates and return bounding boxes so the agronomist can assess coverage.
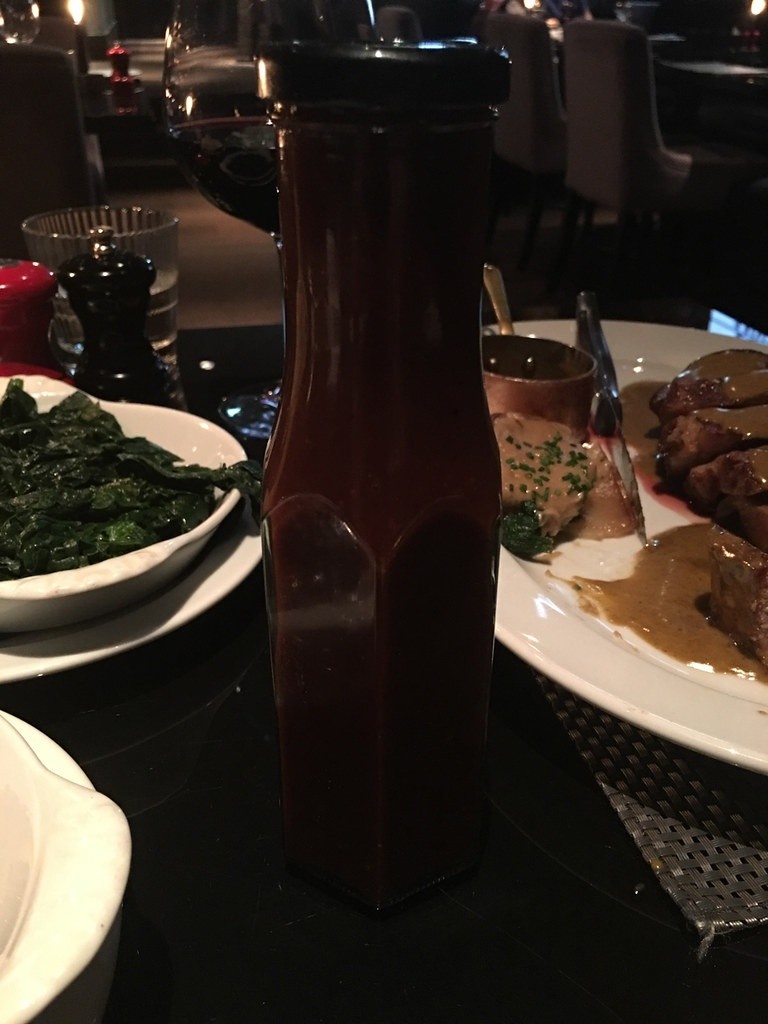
[0,494,262,683]
[482,319,768,779]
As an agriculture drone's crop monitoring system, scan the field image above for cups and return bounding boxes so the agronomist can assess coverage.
[21,205,180,360]
[479,333,597,432]
[0,0,42,45]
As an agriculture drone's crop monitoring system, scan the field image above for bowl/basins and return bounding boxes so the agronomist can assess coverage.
[0,376,246,634]
[0,708,132,1024]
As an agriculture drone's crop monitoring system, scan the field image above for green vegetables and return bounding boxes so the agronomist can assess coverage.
[0,376,265,583]
[501,500,555,559]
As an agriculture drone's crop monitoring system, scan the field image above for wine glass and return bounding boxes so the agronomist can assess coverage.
[164,0,377,438]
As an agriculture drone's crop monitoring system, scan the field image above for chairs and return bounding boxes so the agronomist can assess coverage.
[548,20,768,321]
[0,44,94,263]
[484,13,595,272]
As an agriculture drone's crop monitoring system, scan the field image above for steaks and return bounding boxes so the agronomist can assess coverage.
[490,412,594,537]
[648,348,768,669]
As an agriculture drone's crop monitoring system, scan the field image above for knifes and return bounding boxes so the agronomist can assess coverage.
[578,291,647,543]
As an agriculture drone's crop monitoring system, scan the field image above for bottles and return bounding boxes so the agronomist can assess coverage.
[58,225,168,398]
[0,258,58,367]
[106,41,134,115]
[256,35,509,905]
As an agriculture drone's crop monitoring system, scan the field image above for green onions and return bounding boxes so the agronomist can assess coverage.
[505,430,596,506]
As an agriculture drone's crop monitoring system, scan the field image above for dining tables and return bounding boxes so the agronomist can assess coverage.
[0,324,768,1024]
[656,60,768,151]
